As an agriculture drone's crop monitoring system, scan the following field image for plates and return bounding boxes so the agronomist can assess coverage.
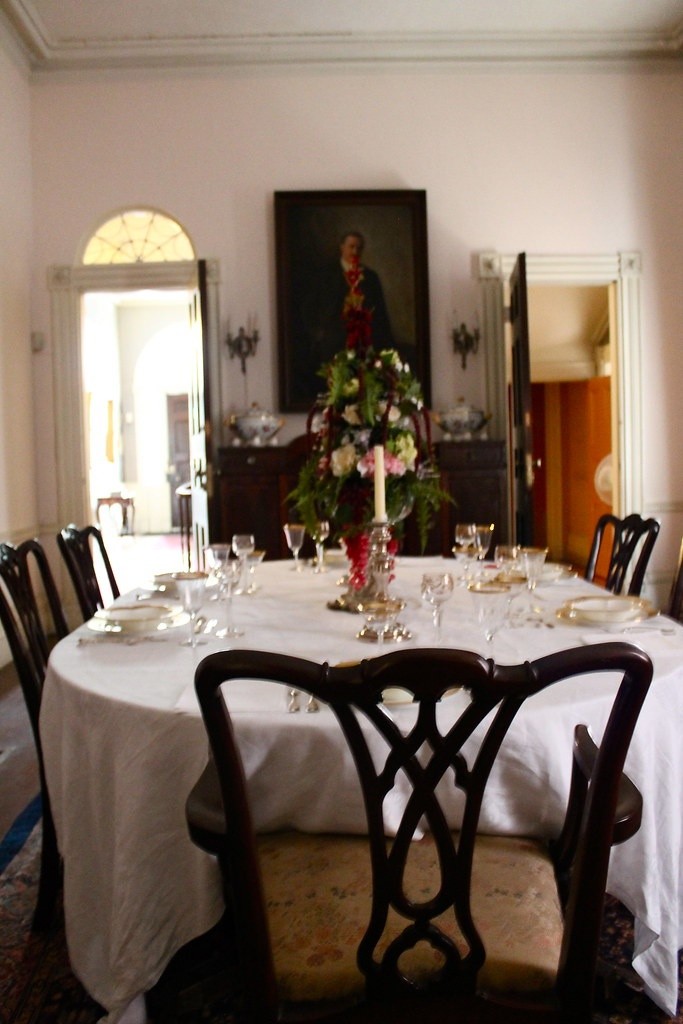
[86,612,189,636]
[336,661,462,706]
[554,600,660,629]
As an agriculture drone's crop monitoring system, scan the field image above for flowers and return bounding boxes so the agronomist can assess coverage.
[284,349,457,558]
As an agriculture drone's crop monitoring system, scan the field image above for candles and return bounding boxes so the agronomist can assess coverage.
[371,446,389,524]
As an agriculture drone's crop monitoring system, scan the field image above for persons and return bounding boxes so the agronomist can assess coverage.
[298,232,396,402]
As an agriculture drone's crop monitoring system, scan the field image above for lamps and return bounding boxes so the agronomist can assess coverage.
[451,323,480,371]
[225,328,258,375]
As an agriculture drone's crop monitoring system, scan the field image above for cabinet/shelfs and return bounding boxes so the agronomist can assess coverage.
[216,442,509,561]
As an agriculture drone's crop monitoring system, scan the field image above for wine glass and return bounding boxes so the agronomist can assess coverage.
[233,535,265,595]
[467,583,511,662]
[283,523,306,573]
[357,596,406,657]
[420,572,455,640]
[309,519,329,573]
[173,572,210,646]
[202,543,231,605]
[451,523,548,615]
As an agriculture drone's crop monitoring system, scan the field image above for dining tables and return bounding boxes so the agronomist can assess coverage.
[39,551,683,1024]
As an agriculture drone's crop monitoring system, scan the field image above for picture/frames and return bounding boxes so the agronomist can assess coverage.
[271,187,433,413]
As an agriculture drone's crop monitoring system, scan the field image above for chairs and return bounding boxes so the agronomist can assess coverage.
[57,525,120,622]
[143,641,652,1023]
[0,544,69,768]
[584,514,662,597]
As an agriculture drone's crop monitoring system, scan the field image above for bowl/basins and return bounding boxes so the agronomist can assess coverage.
[94,605,172,631]
[571,597,638,622]
[154,572,176,597]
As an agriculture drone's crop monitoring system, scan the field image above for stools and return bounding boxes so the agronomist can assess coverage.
[97,497,135,535]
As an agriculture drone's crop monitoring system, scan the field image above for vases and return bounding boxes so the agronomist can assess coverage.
[338,524,398,590]
